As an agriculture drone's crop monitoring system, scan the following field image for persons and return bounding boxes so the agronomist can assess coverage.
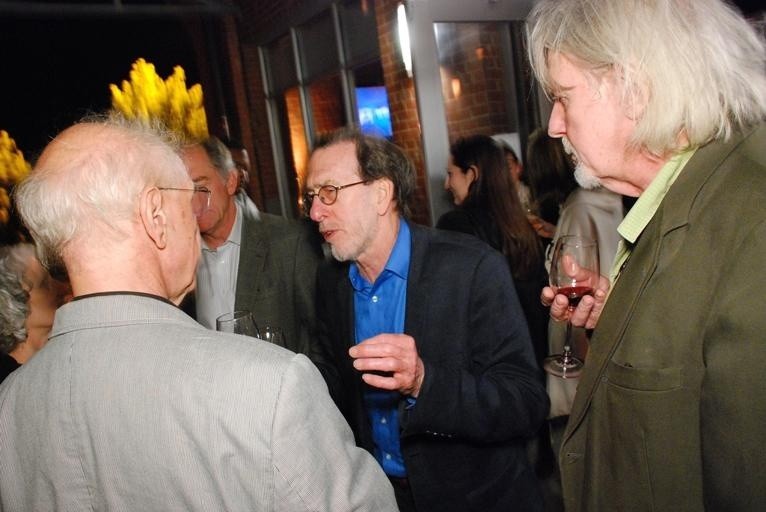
[1,110,398,509]
[175,139,325,359]
[436,127,639,420]
[306,127,562,511]
[526,0,765,512]
[0,243,72,385]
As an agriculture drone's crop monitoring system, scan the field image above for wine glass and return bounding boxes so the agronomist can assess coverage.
[541,235,599,379]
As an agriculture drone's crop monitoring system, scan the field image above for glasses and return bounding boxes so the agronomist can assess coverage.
[304,180,368,205]
[157,186,210,216]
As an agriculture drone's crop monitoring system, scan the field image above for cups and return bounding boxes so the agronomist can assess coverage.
[216,310,287,354]
